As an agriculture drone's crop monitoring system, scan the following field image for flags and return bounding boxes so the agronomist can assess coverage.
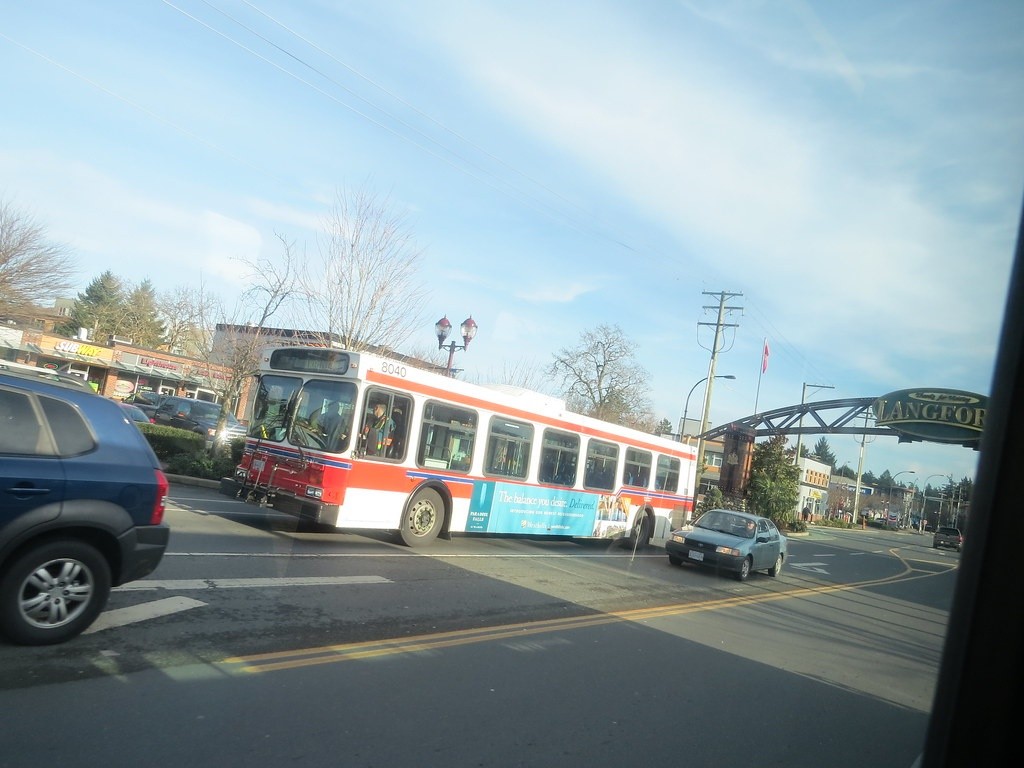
[763,342,769,374]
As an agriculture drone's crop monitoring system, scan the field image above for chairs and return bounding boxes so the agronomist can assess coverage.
[719,518,732,533]
[541,459,662,490]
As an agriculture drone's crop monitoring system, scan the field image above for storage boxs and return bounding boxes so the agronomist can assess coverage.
[424,458,448,468]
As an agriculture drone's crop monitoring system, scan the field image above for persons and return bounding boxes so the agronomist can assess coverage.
[317,402,346,452]
[739,521,755,539]
[364,403,396,457]
[802,505,812,521]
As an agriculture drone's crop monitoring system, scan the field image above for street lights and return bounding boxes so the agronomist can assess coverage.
[435,314,479,376]
[886,471,915,526]
[922,474,945,522]
[680,375,738,442]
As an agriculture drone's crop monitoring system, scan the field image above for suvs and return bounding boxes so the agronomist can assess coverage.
[156,395,248,445]
[0,358,173,647]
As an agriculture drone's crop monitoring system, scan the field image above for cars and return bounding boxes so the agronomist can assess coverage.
[119,403,156,425]
[120,392,167,423]
[665,509,789,582]
[932,526,962,552]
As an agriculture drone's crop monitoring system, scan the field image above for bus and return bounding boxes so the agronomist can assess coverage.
[216,344,703,550]
